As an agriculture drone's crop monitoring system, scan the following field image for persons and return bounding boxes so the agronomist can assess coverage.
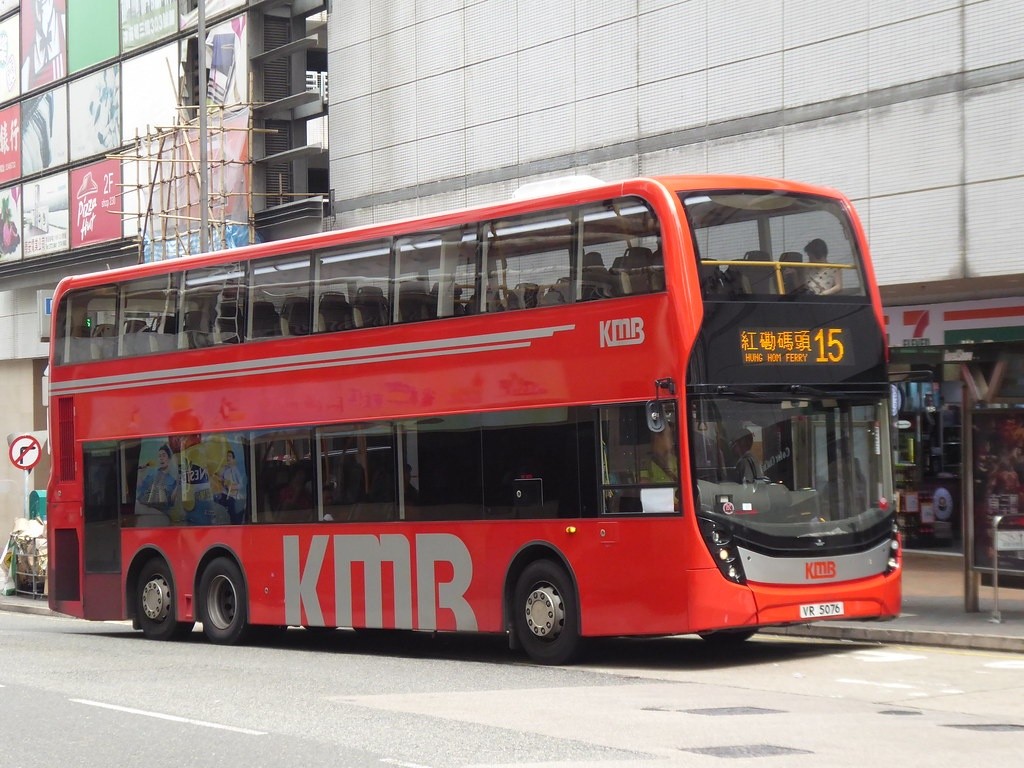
[138,444,180,518]
[280,467,311,512]
[220,450,242,495]
[784,239,843,297]
[822,437,867,520]
[631,421,679,511]
[729,429,761,486]
[584,252,609,299]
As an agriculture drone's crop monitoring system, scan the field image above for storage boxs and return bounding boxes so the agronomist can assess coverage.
[920,502,935,524]
[934,522,953,538]
[904,491,918,512]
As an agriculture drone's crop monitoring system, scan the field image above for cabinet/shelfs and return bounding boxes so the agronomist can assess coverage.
[940,403,963,468]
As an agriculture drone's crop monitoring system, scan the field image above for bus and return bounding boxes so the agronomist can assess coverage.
[49,175,933,663]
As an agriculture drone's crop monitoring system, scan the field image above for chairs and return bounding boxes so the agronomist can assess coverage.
[184,310,210,348]
[123,319,149,354]
[585,248,609,299]
[740,249,777,295]
[92,324,114,358]
[213,304,243,343]
[431,280,464,316]
[610,256,627,297]
[319,290,354,330]
[723,258,746,292]
[487,277,570,313]
[651,250,664,292]
[150,316,177,352]
[252,301,281,339]
[775,251,809,297]
[700,257,729,296]
[401,278,430,321]
[279,296,309,336]
[617,247,653,291]
[353,286,388,328]
